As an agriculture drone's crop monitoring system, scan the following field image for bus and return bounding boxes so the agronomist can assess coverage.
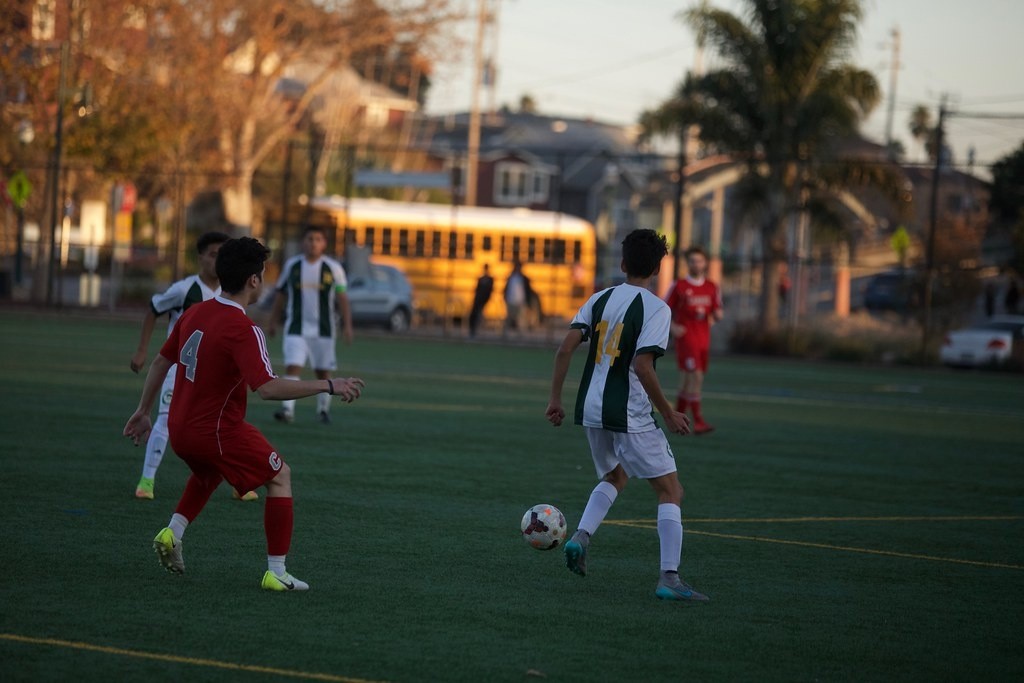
[300,196,599,336]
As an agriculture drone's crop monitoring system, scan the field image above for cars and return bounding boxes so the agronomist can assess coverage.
[843,245,1024,375]
[336,259,413,335]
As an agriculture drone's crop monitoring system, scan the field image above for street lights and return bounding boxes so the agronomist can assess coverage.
[911,92,952,369]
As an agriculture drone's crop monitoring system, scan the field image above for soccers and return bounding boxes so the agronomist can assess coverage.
[520,503,567,552]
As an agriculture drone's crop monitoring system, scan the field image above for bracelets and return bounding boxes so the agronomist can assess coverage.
[327,379,334,395]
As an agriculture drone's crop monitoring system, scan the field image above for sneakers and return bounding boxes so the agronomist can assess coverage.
[562,530,590,576]
[262,570,310,590]
[152,528,186,576]
[232,487,258,500]
[136,475,154,499]
[656,579,709,600]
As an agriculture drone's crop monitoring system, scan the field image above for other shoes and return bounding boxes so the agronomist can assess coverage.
[693,421,714,435]
[317,411,329,423]
[274,407,292,424]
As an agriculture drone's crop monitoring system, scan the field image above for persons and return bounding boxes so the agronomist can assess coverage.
[129,232,259,502]
[662,246,724,435]
[503,262,531,343]
[269,226,354,425]
[468,264,495,339]
[123,235,366,591]
[544,229,709,603]
